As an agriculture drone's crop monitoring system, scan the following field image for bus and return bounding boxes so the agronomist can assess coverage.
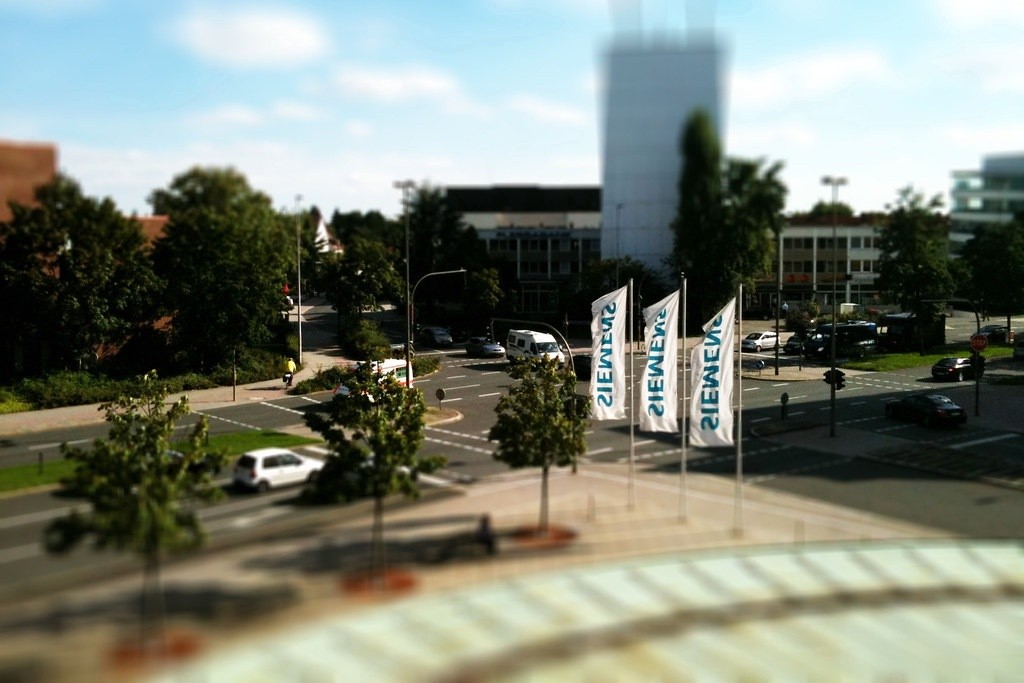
[805,320,877,361]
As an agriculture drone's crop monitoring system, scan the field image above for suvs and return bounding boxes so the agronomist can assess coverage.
[231,447,326,494]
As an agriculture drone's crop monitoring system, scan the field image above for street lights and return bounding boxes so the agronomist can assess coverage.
[406,267,469,411]
[821,175,849,437]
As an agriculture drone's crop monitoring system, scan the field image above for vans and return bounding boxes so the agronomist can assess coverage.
[506,328,566,371]
[742,331,782,352]
[328,358,414,404]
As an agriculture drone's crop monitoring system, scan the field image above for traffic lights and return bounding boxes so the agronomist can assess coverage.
[977,355,987,378]
[485,323,494,344]
[836,370,846,390]
[969,355,976,367]
[409,340,416,359]
[823,370,832,385]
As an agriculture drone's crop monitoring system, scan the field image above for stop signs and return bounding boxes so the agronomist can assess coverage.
[971,333,989,352]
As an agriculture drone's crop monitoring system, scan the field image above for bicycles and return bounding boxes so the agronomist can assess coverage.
[284,374,290,391]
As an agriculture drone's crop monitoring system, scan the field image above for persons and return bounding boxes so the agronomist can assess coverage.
[283,357,296,386]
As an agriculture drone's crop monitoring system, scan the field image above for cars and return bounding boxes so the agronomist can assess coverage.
[975,324,1024,359]
[931,356,974,382]
[466,337,505,359]
[420,325,453,348]
[783,330,806,355]
[568,355,593,382]
[885,393,968,427]
